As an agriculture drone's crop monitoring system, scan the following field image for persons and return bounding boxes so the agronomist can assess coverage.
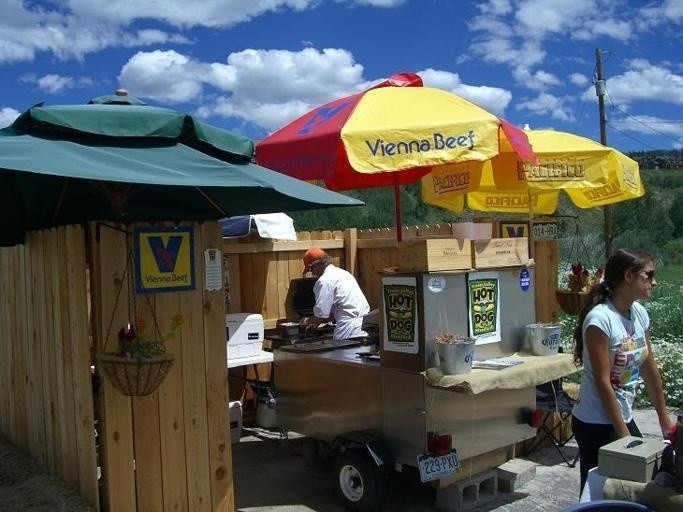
[299,247,370,339]
[571,246,677,501]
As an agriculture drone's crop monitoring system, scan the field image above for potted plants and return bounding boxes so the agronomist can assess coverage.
[524,308,565,354]
[428,328,477,374]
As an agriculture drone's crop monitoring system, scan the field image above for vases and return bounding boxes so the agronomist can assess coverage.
[555,294,596,316]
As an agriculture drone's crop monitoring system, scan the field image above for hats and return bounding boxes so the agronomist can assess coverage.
[303,248,324,277]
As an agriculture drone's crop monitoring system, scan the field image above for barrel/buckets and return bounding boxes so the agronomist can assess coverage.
[452,222,492,241]
[526,324,563,356]
[437,336,478,375]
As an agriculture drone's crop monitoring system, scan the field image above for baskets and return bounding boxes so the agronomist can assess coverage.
[554,292,592,315]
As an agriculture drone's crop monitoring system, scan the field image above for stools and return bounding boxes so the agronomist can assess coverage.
[525,404,581,469]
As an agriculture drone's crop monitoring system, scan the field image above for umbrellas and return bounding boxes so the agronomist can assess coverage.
[420,122,647,221]
[256,72,538,242]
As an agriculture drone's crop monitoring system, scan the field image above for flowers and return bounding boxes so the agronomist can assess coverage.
[106,308,186,358]
[557,260,606,295]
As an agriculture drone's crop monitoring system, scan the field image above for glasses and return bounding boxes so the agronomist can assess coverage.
[643,269,656,277]
